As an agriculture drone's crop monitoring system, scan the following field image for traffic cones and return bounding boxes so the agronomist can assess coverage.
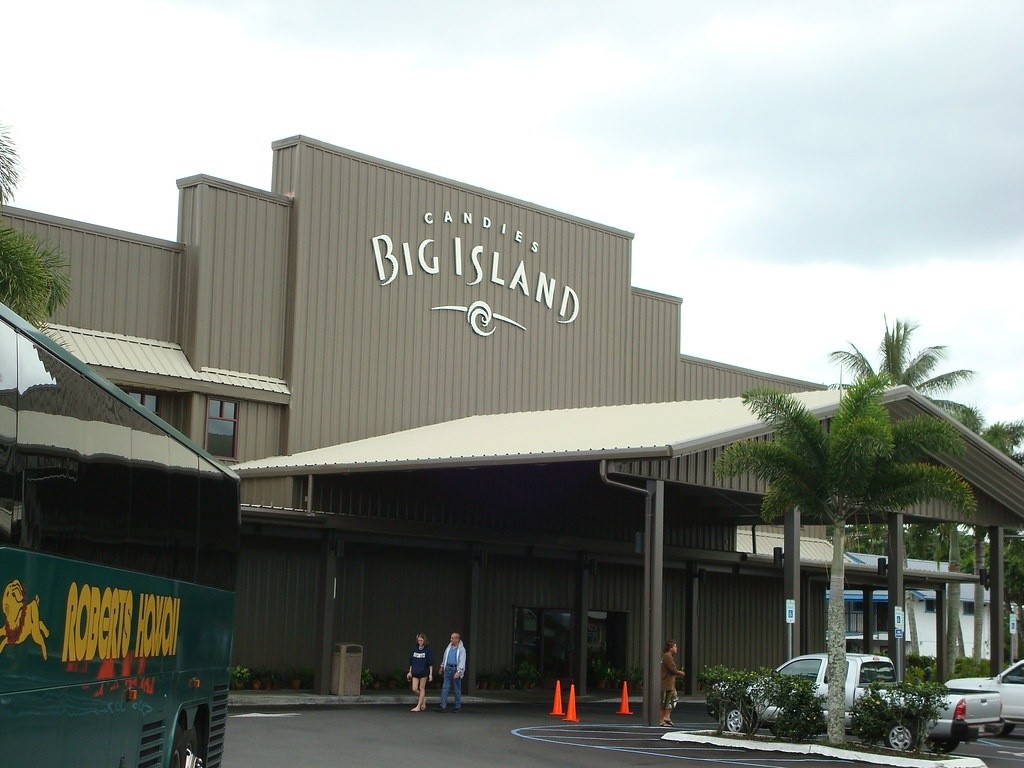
[562,684,579,722]
[616,682,635,715]
[550,679,566,716]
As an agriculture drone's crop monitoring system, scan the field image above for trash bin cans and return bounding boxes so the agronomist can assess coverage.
[330,642,363,696]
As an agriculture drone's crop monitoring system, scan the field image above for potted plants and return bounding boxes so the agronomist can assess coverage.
[591,659,685,692]
[477,662,541,691]
[227,666,407,692]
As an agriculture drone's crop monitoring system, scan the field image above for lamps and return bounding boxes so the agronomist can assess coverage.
[877,558,888,575]
[693,568,706,585]
[979,568,990,591]
[773,546,784,568]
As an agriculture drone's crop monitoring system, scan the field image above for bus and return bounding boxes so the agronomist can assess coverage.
[0,302,241,768]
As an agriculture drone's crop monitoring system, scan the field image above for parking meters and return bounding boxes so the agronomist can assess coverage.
[895,610,904,684]
[1009,614,1017,667]
[785,600,796,661]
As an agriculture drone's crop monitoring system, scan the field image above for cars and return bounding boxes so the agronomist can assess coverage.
[944,658,1024,737]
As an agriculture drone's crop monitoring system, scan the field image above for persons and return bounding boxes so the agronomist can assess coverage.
[407,633,433,712]
[661,641,685,726]
[439,633,466,712]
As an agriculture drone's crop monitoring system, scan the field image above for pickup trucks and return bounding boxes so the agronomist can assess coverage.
[704,652,1002,754]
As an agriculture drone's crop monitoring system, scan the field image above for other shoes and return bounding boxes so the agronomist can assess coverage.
[421,698,426,710]
[411,708,420,711]
[452,708,461,712]
[435,707,446,712]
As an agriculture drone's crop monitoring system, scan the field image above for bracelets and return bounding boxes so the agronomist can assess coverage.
[457,671,460,673]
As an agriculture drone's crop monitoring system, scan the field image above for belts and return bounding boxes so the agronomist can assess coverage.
[447,664,457,667]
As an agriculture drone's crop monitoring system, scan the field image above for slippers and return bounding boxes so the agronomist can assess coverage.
[664,719,674,726]
[659,722,671,727]
